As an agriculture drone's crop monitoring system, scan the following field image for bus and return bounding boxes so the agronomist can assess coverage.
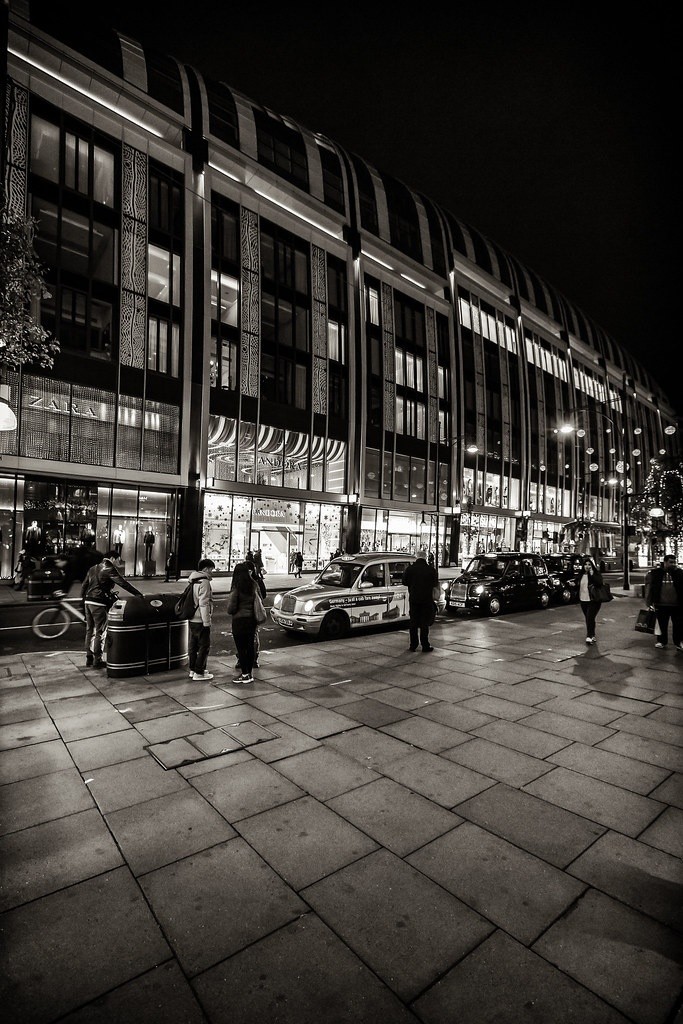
[558,520,639,573]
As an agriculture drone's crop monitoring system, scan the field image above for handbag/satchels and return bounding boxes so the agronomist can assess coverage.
[432,582,441,601]
[649,606,662,636]
[635,608,657,634]
[101,591,120,613]
[254,583,267,621]
[587,580,614,603]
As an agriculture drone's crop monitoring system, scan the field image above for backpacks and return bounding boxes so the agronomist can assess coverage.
[175,576,211,620]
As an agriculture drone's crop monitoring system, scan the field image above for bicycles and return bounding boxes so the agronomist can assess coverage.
[32,590,87,640]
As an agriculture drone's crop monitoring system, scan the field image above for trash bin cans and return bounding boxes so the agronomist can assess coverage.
[629,560,634,571]
[106,593,188,678]
[28,570,65,600]
[599,561,605,573]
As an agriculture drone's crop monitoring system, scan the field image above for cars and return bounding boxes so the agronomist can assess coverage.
[442,551,553,617]
[270,551,447,641]
[541,553,591,605]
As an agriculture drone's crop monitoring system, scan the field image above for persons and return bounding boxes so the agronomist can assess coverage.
[26,520,41,558]
[81,551,145,669]
[481,537,494,552]
[330,548,345,573]
[113,524,125,560]
[290,548,304,578]
[227,549,267,684]
[574,559,603,644]
[467,479,555,513]
[646,554,683,650]
[143,526,155,561]
[403,551,439,653]
[185,559,214,680]
[44,553,98,598]
[164,552,180,582]
[82,523,96,548]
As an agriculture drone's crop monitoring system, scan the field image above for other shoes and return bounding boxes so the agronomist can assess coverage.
[252,661,259,668]
[586,637,592,643]
[93,662,107,669]
[189,669,209,677]
[674,642,683,650]
[193,672,213,680]
[409,646,417,652]
[86,658,94,667]
[655,642,665,648]
[248,675,254,681]
[235,660,241,669]
[232,673,251,683]
[592,637,597,642]
[422,647,434,653]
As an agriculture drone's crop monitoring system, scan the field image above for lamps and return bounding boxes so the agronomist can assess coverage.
[453,434,478,453]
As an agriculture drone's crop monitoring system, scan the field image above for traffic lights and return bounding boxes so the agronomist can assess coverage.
[543,532,547,543]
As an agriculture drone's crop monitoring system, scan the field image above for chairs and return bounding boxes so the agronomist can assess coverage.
[393,564,404,578]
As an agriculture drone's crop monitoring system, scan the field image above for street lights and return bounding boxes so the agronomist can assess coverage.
[420,435,478,574]
[561,408,664,590]
[581,469,618,521]
